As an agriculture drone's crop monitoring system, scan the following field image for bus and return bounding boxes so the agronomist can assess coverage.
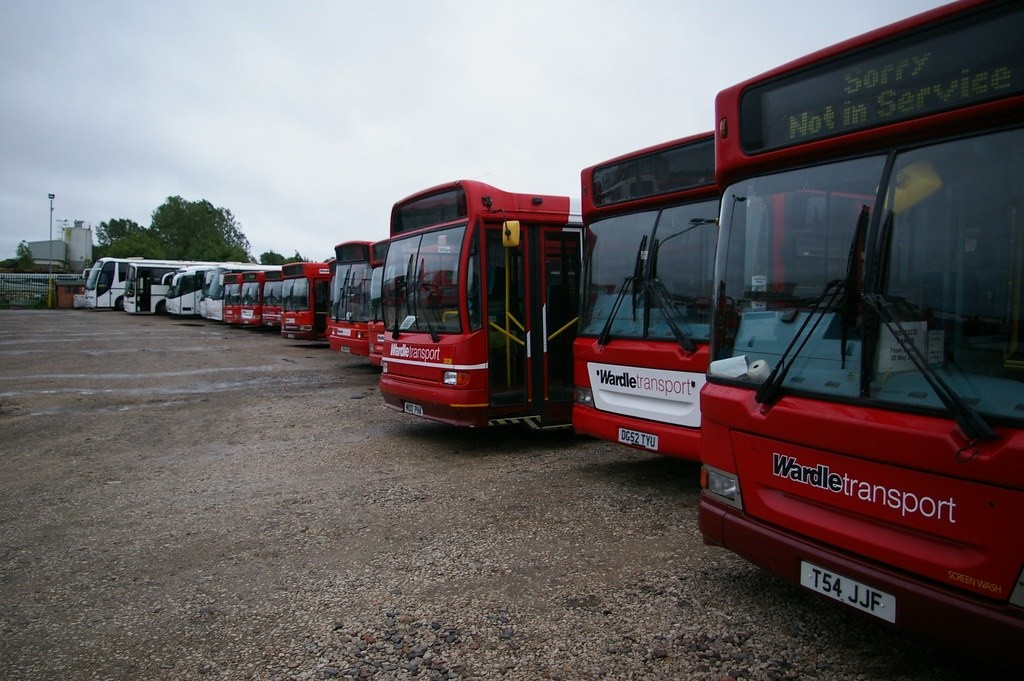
[379,180,580,432]
[571,130,879,467]
[81,256,146,311]
[700,0,1024,663]
[162,240,391,371]
[123,263,186,317]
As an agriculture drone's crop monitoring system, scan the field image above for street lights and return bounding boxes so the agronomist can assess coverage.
[48,194,55,308]
